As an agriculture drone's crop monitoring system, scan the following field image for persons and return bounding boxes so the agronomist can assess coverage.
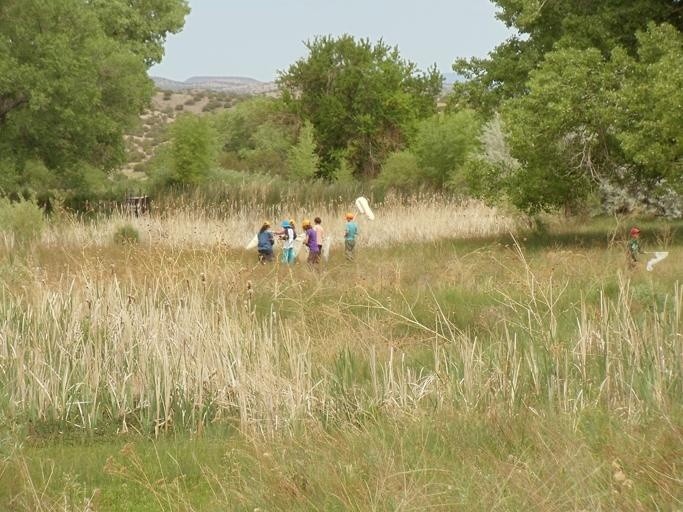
[278,219,297,264]
[625,228,645,272]
[313,217,325,263]
[302,219,320,264]
[342,213,359,260]
[257,220,275,263]
[271,219,296,234]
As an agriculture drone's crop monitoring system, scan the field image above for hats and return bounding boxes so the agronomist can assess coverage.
[344,213,354,220]
[262,219,312,227]
